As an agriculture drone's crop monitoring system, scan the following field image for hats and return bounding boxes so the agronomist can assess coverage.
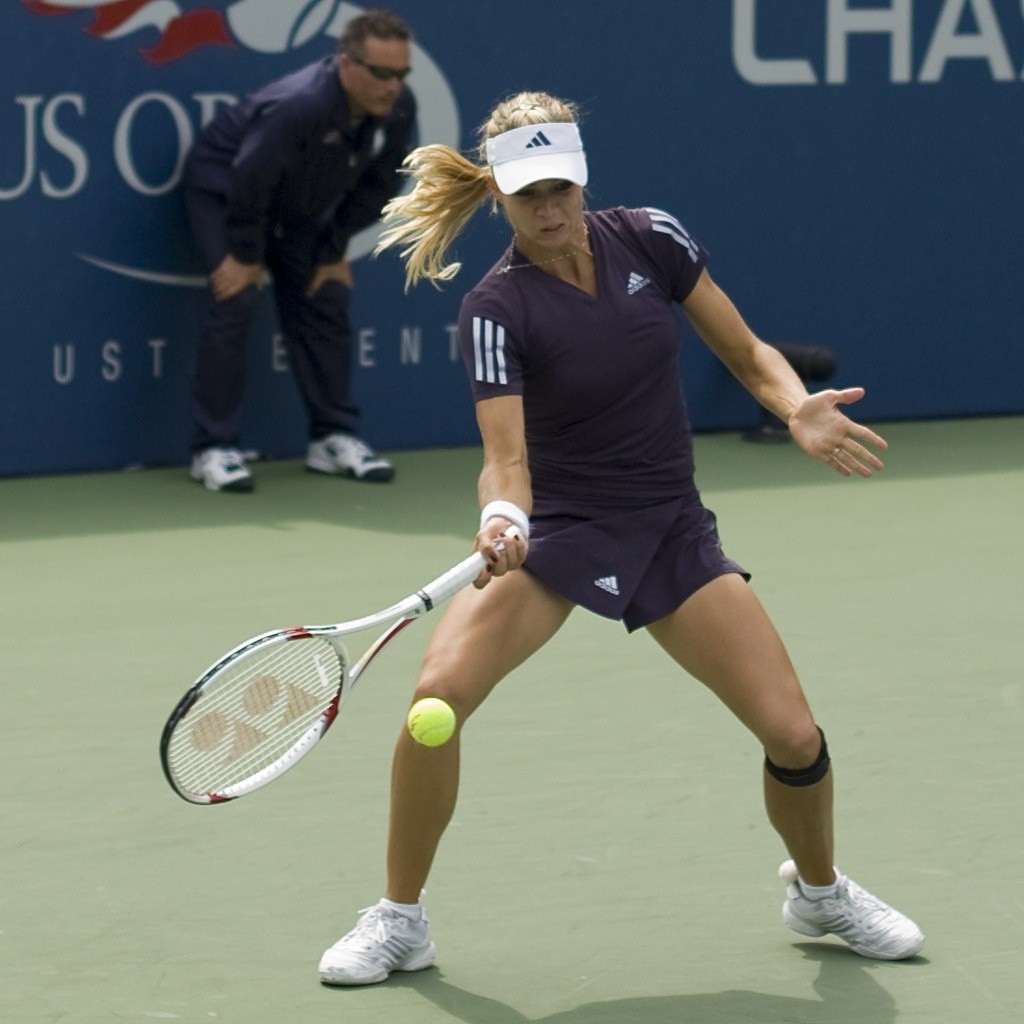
[485,122,589,195]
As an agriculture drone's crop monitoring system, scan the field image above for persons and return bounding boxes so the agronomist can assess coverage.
[315,92,927,982]
[179,12,418,492]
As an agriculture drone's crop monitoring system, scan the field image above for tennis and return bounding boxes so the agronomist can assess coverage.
[406,697,458,749]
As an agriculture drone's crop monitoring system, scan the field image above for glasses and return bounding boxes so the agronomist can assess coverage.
[346,50,412,82]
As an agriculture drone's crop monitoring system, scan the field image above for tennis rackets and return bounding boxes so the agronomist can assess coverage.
[160,525,525,807]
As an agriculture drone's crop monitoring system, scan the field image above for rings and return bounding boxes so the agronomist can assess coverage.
[226,281,231,288]
[832,446,841,456]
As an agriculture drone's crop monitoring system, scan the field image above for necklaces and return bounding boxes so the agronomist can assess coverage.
[496,222,587,275]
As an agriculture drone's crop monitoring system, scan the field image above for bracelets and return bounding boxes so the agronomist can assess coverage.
[480,501,529,539]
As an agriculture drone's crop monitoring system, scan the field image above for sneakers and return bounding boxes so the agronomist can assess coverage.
[189,446,257,492]
[778,858,926,960]
[304,433,396,481]
[319,888,435,984]
